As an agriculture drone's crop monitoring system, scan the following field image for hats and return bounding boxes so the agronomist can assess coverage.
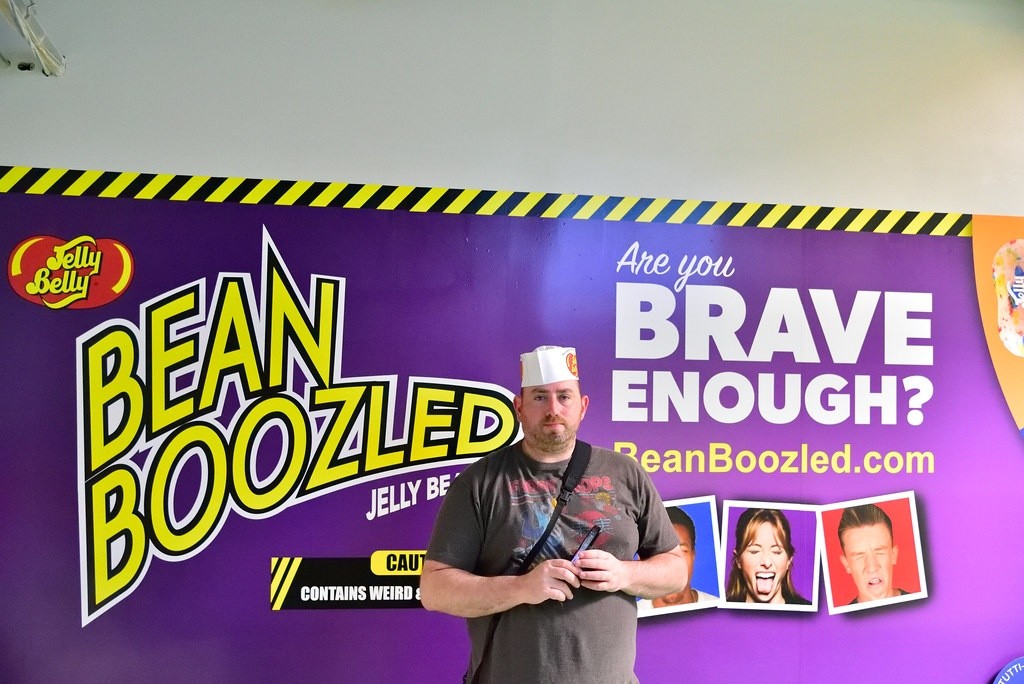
[520,344,579,388]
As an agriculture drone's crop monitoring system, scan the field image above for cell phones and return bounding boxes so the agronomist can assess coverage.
[571,526,602,563]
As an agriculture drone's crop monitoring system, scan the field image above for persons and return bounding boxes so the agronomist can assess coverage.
[725,508,812,605]
[419,344,689,684]
[839,504,910,604]
[636,507,718,612]
